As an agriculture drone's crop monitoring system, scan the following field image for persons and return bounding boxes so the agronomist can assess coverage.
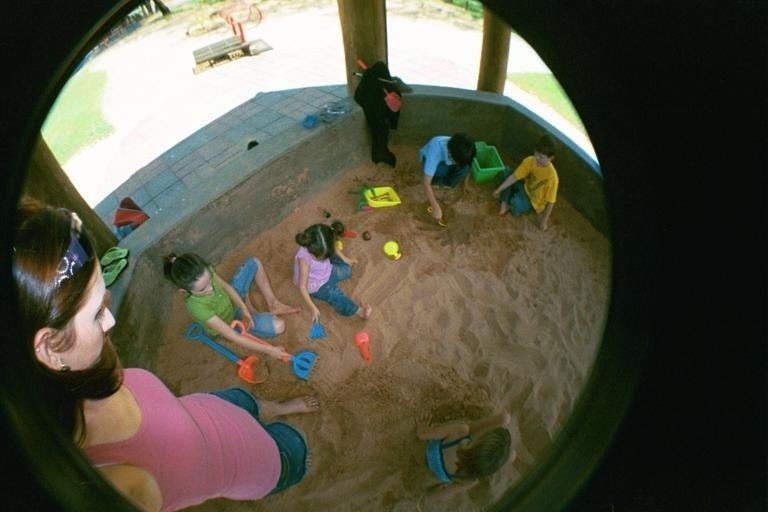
[491,131,561,233]
[412,405,520,505]
[6,193,322,511]
[291,221,372,325]
[160,249,301,364]
[415,130,478,224]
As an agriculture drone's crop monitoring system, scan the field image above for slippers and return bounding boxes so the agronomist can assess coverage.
[101,246,129,265]
[101,258,128,287]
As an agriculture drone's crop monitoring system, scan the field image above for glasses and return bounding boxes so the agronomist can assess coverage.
[53,205,94,287]
